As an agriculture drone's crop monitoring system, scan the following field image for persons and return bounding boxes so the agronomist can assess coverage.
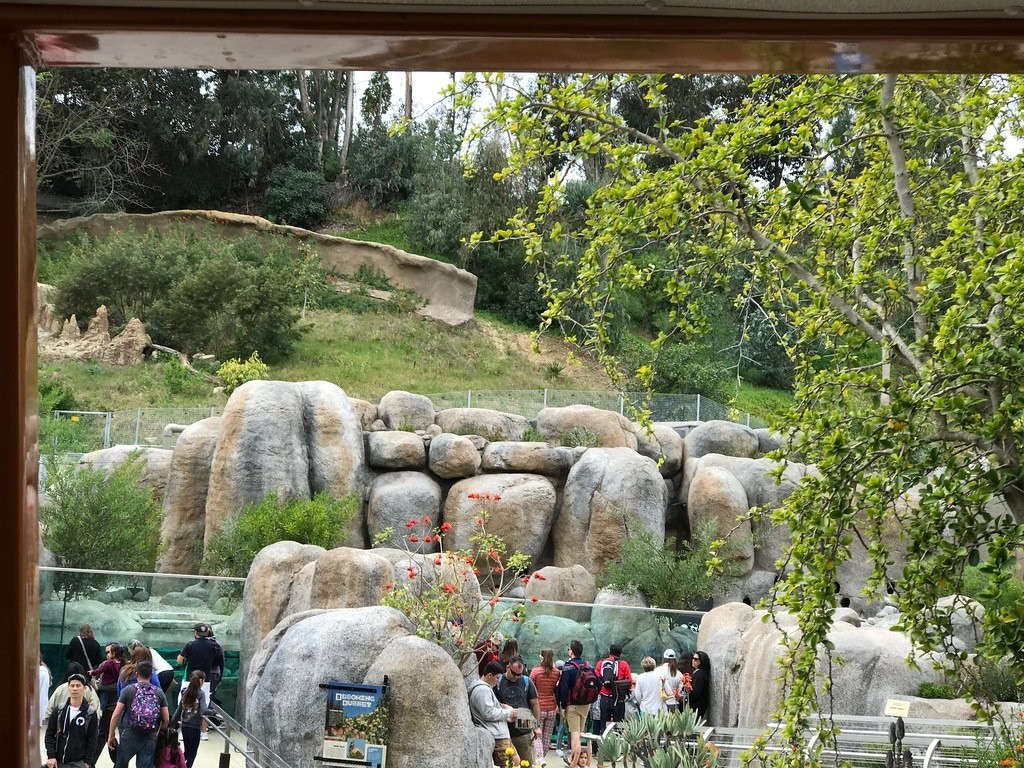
[146,645,174,692]
[128,640,174,693]
[40,655,51,727]
[475,632,525,675]
[532,641,638,768]
[108,662,169,768]
[468,661,521,768]
[66,624,103,672]
[154,727,187,768]
[655,649,684,713]
[169,670,223,768]
[678,651,694,675]
[45,674,99,768]
[570,732,592,768]
[45,662,100,720]
[117,647,161,697]
[636,657,669,715]
[86,642,127,768]
[493,657,541,768]
[683,651,711,719]
[177,623,226,742]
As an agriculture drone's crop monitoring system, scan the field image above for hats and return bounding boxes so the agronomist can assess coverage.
[492,632,504,646]
[663,648,676,659]
[555,659,565,668]
[68,673,87,686]
[194,623,208,638]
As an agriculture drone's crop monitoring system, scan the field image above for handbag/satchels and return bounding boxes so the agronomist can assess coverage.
[91,673,102,690]
[612,679,631,697]
[525,735,544,766]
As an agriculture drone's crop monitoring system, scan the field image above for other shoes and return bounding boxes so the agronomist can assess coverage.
[200,731,210,741]
[178,732,185,742]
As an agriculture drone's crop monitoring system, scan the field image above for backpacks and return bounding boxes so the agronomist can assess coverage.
[601,658,621,690]
[125,683,161,736]
[568,660,599,705]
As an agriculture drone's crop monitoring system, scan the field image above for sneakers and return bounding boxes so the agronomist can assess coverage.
[556,749,564,757]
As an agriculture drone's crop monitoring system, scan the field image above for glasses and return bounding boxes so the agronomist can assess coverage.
[568,649,572,651]
[691,657,701,661]
[105,651,111,655]
[509,667,522,676]
